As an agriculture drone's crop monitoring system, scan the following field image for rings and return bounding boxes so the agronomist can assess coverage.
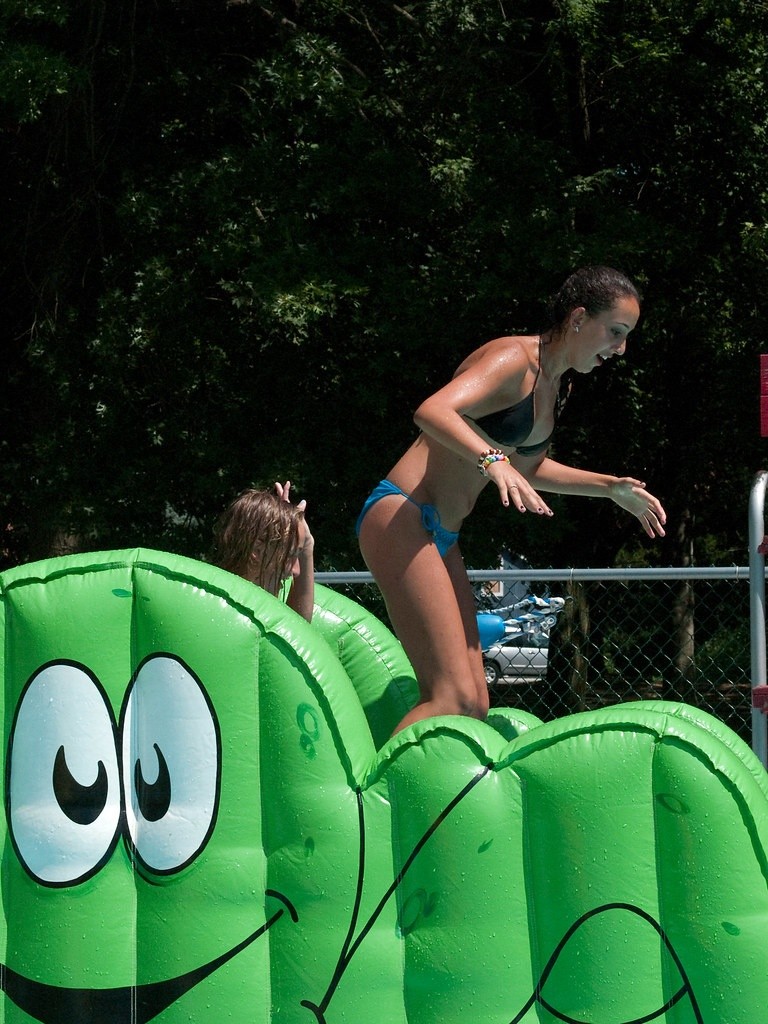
[507,484,519,493]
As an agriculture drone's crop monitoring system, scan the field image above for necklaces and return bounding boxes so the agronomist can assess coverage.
[542,333,564,406]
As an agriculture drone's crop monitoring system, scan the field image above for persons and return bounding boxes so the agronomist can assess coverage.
[206,478,316,624]
[354,264,667,739]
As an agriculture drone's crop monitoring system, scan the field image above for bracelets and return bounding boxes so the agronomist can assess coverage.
[479,454,511,476]
[477,448,505,468]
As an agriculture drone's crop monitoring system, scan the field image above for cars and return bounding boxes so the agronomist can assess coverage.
[480,626,550,686]
